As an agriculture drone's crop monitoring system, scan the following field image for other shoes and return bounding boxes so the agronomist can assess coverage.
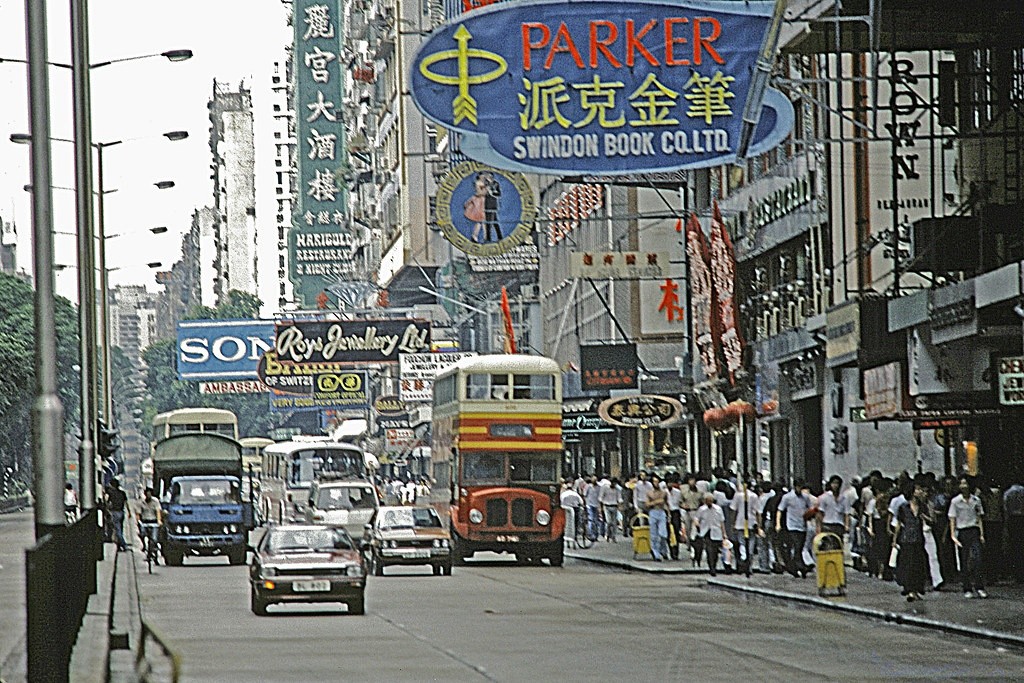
[965,592,974,599]
[978,590,988,598]
[907,596,916,602]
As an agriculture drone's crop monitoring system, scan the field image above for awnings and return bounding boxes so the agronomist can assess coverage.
[562,398,615,433]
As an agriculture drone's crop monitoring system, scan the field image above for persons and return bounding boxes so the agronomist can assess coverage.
[104,479,132,551]
[65,485,78,523]
[474,455,500,478]
[560,467,1024,602]
[320,489,372,509]
[182,484,217,502]
[376,473,433,503]
[463,173,505,243]
[134,490,163,553]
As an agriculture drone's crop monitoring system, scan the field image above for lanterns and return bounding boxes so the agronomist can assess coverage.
[727,400,754,423]
[703,408,736,430]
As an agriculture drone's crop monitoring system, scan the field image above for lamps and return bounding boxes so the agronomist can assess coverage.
[771,289,780,298]
[787,283,795,292]
[815,347,821,356]
[783,366,789,374]
[796,276,808,287]
[763,293,770,301]
[807,351,816,358]
[824,262,845,276]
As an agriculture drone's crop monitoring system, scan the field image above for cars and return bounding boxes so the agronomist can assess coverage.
[360,505,453,577]
[242,524,370,615]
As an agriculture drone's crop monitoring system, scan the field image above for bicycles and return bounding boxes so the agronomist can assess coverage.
[576,503,598,550]
[138,520,161,574]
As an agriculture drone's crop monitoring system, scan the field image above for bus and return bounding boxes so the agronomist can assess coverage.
[430,354,566,567]
[261,441,380,526]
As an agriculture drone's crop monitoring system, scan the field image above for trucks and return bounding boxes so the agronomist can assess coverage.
[150,407,276,565]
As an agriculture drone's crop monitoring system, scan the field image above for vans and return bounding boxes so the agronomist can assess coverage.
[306,481,381,541]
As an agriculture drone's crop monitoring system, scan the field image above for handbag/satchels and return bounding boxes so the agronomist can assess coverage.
[669,523,676,547]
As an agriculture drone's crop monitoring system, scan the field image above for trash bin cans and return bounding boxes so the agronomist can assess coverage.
[629,512,652,556]
[812,531,847,597]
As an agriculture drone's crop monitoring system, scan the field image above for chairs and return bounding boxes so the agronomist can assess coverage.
[278,534,296,547]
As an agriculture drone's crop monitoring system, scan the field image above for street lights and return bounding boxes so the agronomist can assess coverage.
[9,130,189,429]
[734,365,751,579]
